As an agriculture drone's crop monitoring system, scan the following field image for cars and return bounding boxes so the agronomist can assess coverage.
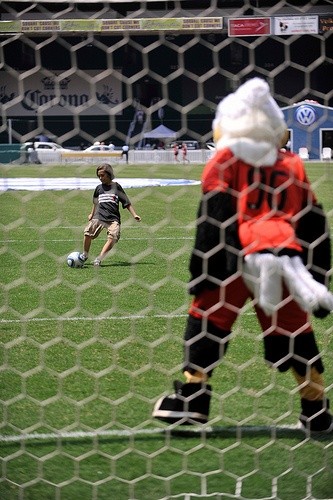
[77,145,120,163]
[20,142,73,163]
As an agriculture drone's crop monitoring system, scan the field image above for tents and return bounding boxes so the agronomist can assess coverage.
[144,125,177,149]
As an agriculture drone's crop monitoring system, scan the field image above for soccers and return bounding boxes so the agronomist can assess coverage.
[66,251,85,268]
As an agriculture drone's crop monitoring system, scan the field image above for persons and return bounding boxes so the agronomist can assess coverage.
[80,163,141,267]
[82,141,190,165]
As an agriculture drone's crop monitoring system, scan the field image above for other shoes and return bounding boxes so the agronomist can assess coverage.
[299,397,332,436]
[94,257,102,266]
[154,377,212,423]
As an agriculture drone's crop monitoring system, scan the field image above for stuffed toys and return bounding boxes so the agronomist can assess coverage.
[153,78,333,437]
